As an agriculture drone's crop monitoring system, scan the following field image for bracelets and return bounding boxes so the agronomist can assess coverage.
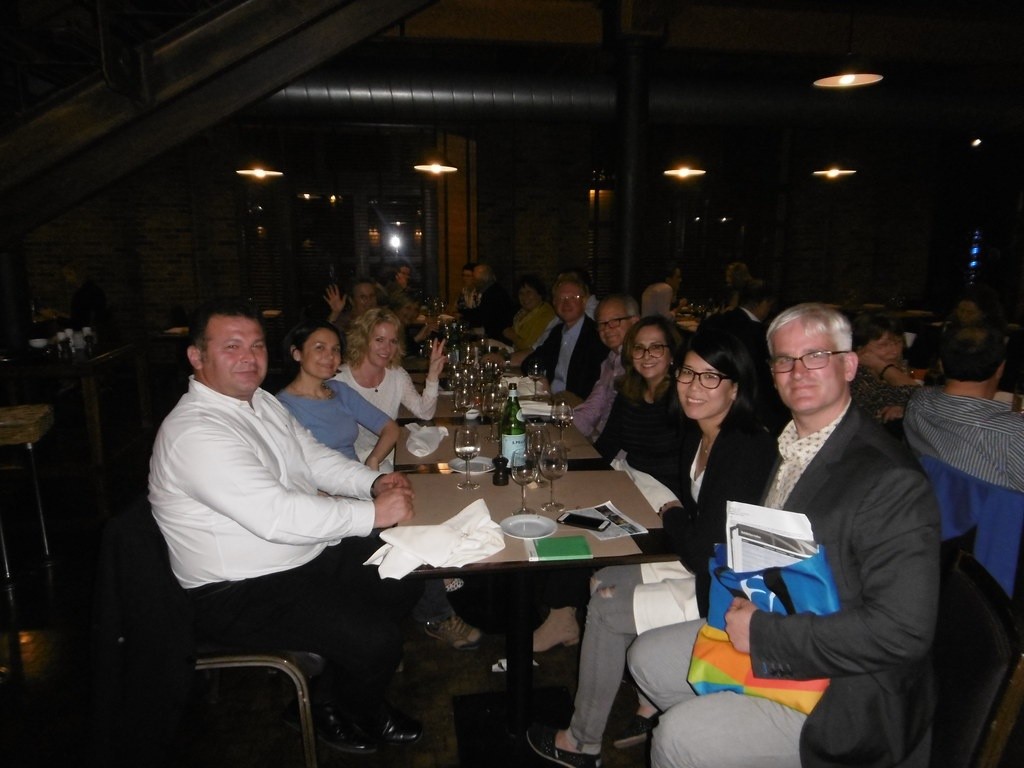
[502,352,511,365]
[880,364,894,378]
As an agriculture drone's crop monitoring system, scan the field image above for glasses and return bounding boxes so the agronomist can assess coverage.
[594,316,633,332]
[673,361,734,390]
[864,340,908,351]
[630,342,670,360]
[765,349,853,373]
[554,293,584,303]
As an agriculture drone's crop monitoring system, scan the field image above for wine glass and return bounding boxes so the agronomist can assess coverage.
[413,292,577,516]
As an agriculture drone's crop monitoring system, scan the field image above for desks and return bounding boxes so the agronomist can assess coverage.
[391,349,683,740]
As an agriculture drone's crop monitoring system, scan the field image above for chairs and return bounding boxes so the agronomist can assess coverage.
[99,498,325,768]
[918,548,1024,768]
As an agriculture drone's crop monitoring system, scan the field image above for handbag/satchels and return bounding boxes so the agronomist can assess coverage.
[686,541,843,717]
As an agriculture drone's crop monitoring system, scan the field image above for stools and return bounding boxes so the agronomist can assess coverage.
[0,401,54,581]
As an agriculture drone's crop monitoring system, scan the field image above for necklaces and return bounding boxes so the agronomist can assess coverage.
[700,440,710,456]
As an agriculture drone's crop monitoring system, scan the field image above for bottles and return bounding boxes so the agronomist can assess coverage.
[57,326,91,359]
[500,383,531,474]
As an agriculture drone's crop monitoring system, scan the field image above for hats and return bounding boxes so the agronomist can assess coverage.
[461,262,477,272]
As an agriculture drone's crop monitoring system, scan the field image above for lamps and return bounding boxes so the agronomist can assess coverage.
[663,156,707,180]
[413,147,458,174]
[813,163,857,180]
[235,160,284,179]
[813,62,884,89]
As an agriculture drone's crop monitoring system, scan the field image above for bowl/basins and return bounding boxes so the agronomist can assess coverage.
[29,338,48,348]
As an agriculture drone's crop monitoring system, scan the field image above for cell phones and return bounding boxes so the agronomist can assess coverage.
[557,512,611,531]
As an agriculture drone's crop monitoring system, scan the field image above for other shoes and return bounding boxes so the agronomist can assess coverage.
[526,724,603,768]
[440,576,464,593]
[611,711,663,749]
[491,636,580,673]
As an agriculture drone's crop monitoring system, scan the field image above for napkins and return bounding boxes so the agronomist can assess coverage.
[507,376,548,398]
[404,423,449,457]
[518,399,552,416]
[362,500,506,580]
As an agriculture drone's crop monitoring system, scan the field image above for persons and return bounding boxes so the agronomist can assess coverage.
[530,315,699,653]
[142,301,425,752]
[329,304,446,474]
[627,304,939,768]
[624,253,1024,493]
[272,316,486,648]
[524,323,779,768]
[439,262,641,447]
[323,260,433,361]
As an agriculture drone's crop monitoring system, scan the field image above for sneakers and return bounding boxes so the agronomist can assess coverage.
[423,613,485,651]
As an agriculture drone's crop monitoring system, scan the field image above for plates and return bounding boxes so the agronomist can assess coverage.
[499,513,558,540]
[447,455,496,475]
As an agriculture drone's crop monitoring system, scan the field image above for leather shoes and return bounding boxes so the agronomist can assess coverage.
[354,702,425,747]
[278,696,380,757]
